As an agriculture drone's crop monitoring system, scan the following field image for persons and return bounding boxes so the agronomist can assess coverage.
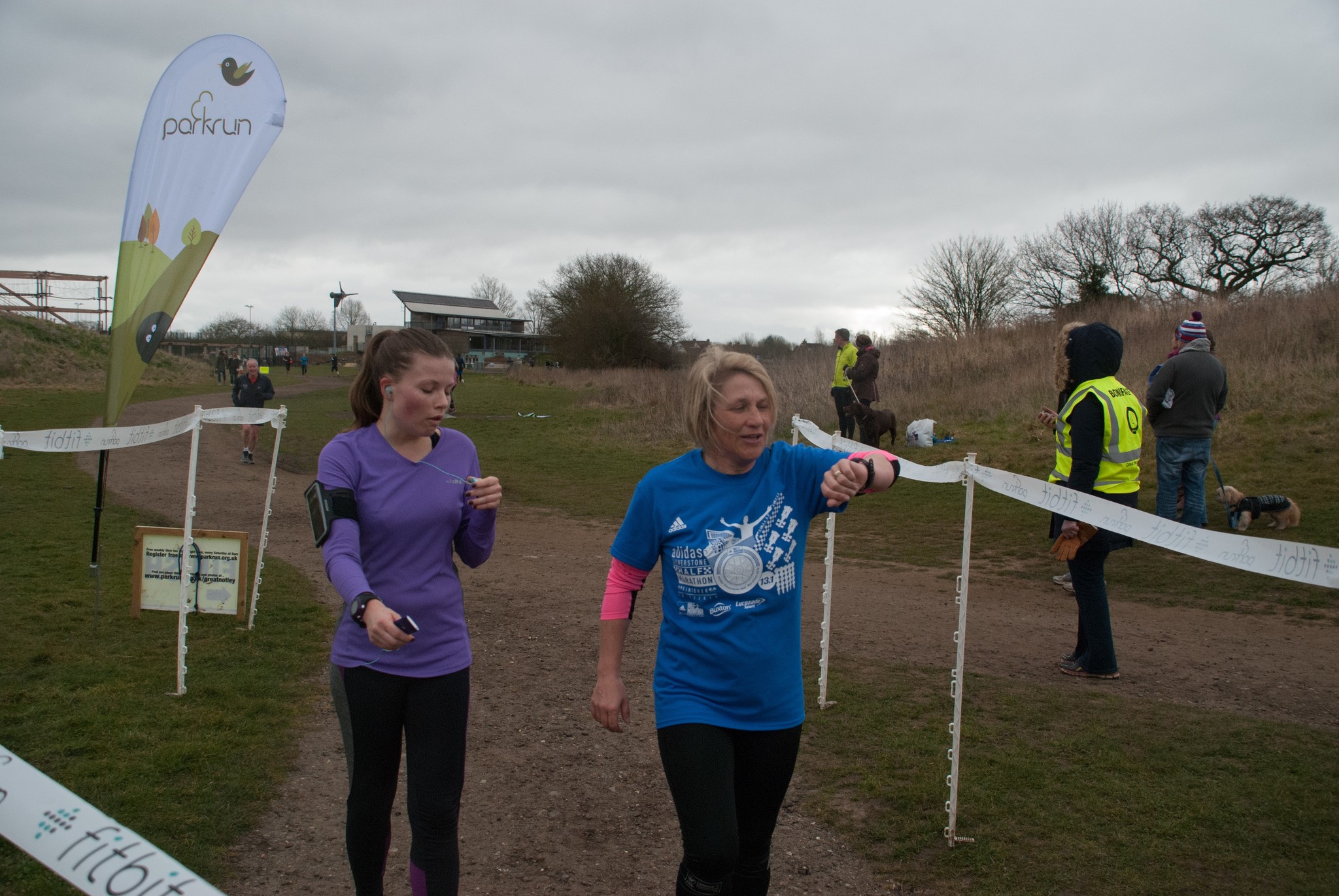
[214,349,245,386]
[1035,322,1149,679]
[445,352,559,415]
[844,334,882,408]
[283,351,292,375]
[300,353,307,376]
[231,358,275,464]
[304,327,502,896]
[1138,311,1229,532]
[331,354,340,376]
[588,345,900,896]
[830,328,859,439]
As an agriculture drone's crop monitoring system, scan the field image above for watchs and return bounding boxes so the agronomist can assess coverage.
[851,457,874,497]
[349,592,382,628]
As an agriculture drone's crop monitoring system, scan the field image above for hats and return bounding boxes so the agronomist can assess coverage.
[855,334,871,347]
[1176,311,1207,343]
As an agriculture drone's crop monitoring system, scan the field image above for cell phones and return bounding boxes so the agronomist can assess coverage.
[394,615,420,634]
[305,481,331,548]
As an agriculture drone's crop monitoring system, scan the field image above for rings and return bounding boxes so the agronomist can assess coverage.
[833,470,842,479]
[1071,531,1075,535]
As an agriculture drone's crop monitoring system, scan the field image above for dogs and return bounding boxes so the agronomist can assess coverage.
[844,402,897,449]
[1214,486,1301,532]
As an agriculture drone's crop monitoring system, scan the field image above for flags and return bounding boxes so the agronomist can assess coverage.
[108,34,285,439]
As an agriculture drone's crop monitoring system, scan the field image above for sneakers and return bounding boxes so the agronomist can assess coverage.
[1052,571,1073,584]
[1062,582,1075,592]
[1060,651,1120,681]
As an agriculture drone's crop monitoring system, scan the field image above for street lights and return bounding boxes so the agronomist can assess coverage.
[244,305,254,344]
[74,302,84,322]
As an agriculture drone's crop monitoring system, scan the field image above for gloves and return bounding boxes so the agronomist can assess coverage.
[1050,521,1099,562]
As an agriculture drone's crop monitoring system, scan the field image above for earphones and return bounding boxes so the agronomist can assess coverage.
[385,386,392,395]
[471,478,478,484]
